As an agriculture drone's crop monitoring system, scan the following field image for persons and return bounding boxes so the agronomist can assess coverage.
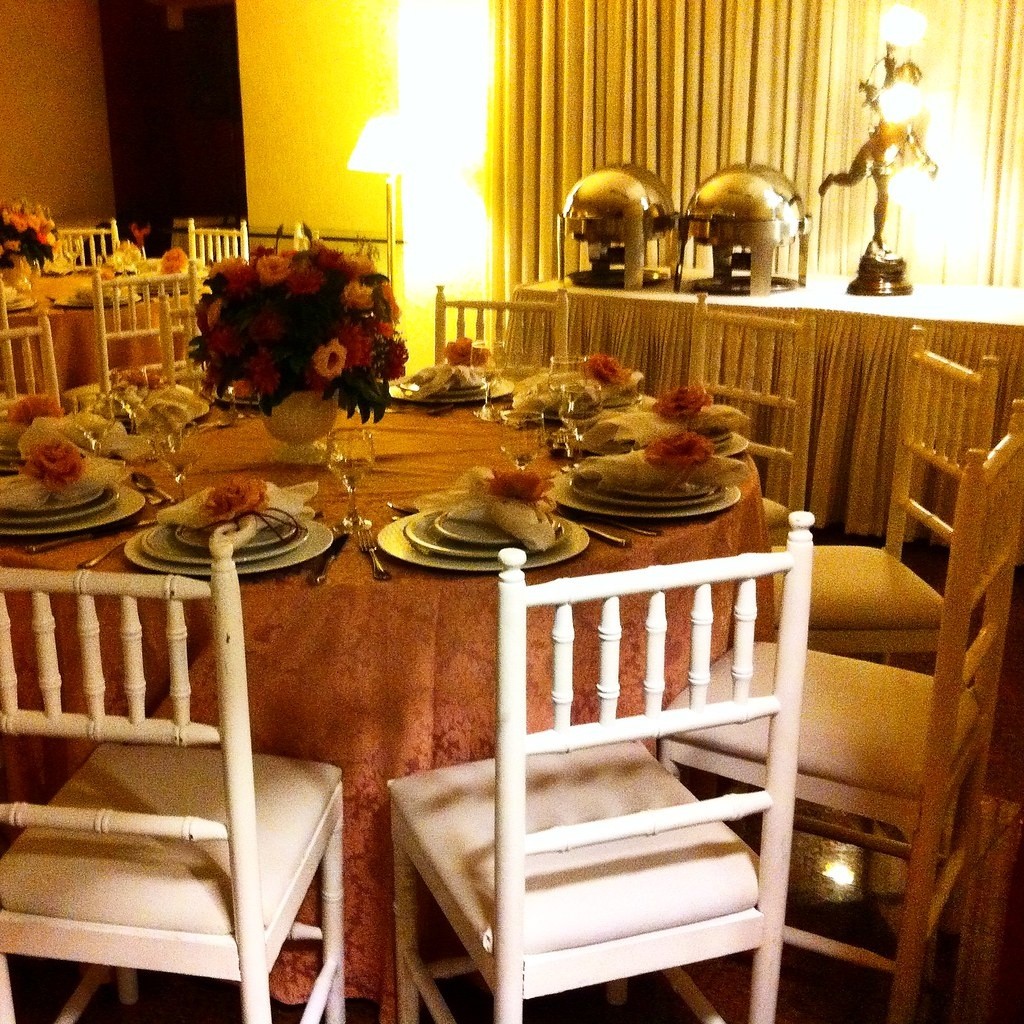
[75,209,150,271]
[817,63,939,251]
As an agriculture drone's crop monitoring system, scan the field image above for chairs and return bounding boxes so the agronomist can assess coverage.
[185,220,252,287]
[765,326,999,673]
[160,293,208,398]
[0,307,62,422]
[435,284,567,382]
[55,218,120,268]
[689,295,817,546]
[1,540,346,1024]
[385,506,814,1024]
[653,401,1024,1024]
[65,261,200,420]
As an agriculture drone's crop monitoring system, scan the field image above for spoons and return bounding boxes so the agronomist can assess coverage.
[133,472,177,504]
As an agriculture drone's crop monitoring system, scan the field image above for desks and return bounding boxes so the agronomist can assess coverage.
[504,275,1024,537]
[0,259,204,392]
[0,374,775,1006]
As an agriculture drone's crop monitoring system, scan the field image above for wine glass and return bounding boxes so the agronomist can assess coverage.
[151,417,204,502]
[471,340,504,421]
[111,364,148,435]
[63,238,82,269]
[549,355,603,472]
[500,410,545,471]
[326,428,375,535]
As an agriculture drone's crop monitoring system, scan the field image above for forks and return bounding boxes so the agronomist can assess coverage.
[357,530,390,580]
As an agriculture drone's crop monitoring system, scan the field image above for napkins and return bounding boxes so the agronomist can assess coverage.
[415,470,564,550]
[586,404,750,441]
[19,425,125,497]
[158,479,319,523]
[575,451,750,491]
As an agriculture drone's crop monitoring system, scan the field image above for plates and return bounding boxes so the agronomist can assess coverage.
[377,432,750,572]
[6,271,209,310]
[213,392,259,405]
[390,376,640,410]
[0,444,335,576]
[114,396,210,429]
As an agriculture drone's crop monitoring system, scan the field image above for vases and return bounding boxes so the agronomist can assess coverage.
[6,250,32,283]
[259,388,337,444]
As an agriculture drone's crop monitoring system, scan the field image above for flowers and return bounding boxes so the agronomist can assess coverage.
[188,226,408,422]
[641,430,714,470]
[6,397,64,421]
[653,387,713,423]
[447,339,488,363]
[585,349,632,384]
[198,477,269,518]
[26,442,84,488]
[489,463,554,504]
[161,246,187,274]
[0,200,54,268]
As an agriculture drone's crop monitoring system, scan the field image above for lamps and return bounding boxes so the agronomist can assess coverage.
[820,5,941,296]
[351,114,444,320]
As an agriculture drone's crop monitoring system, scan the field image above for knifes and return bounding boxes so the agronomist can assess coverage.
[312,533,350,585]
[25,517,157,555]
